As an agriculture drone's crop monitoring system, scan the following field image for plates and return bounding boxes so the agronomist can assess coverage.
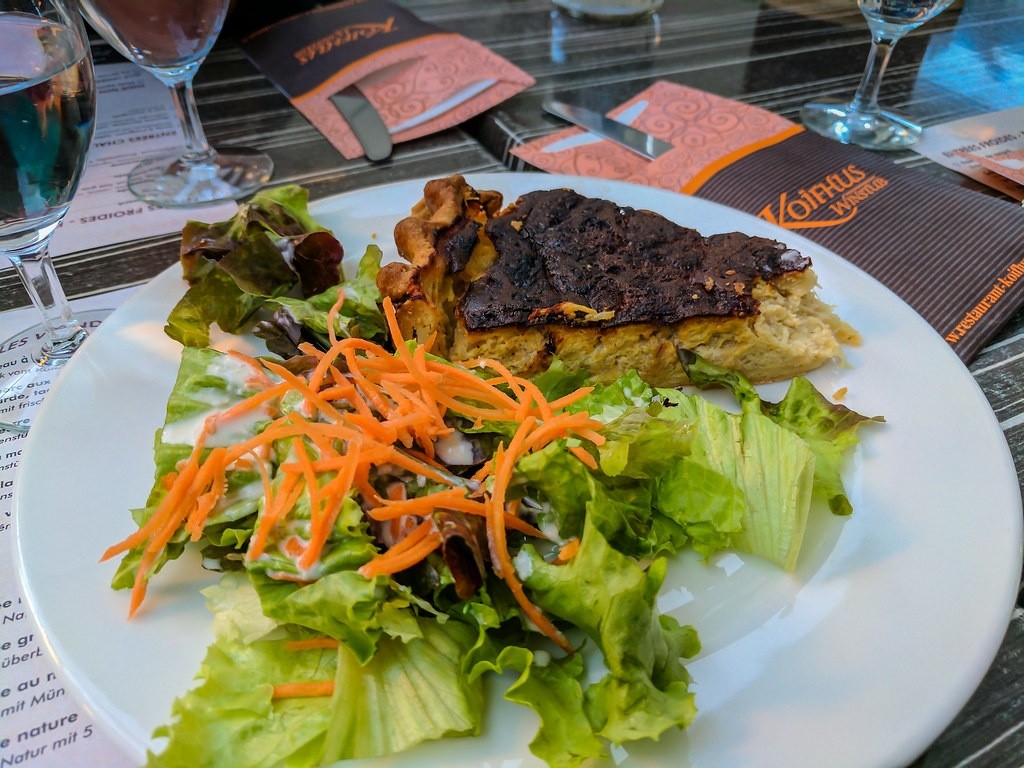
[10,172,1019,768]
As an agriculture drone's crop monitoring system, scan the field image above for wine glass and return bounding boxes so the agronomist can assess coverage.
[72,1,274,209]
[798,1,956,149]
[0,1,118,431]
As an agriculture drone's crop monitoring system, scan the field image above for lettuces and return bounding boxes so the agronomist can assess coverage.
[99,173,886,768]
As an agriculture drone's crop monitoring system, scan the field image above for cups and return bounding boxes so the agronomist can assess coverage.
[547,0,667,25]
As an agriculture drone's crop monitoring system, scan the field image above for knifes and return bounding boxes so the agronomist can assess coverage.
[540,97,676,161]
[329,80,394,164]
